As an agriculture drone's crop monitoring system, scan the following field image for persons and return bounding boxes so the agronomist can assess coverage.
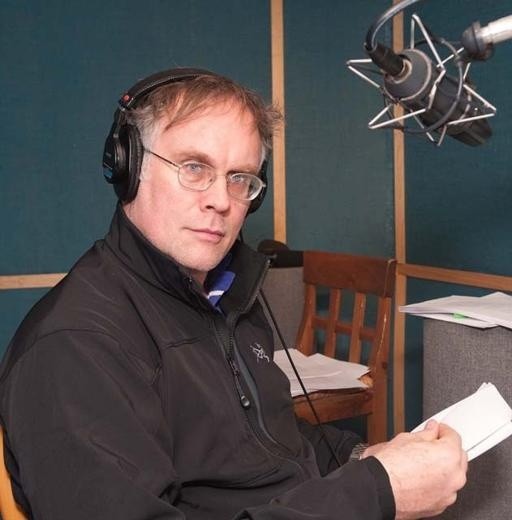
[0,67,471,519]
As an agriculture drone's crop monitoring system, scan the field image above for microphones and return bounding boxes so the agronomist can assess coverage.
[360,36,494,148]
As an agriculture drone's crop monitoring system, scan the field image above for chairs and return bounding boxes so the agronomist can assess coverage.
[294,250,398,445]
[0,426,30,520]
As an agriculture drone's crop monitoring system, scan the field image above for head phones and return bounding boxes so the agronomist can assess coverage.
[101,66,269,217]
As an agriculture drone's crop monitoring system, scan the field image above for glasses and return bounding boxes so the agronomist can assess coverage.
[144,149,267,201]
